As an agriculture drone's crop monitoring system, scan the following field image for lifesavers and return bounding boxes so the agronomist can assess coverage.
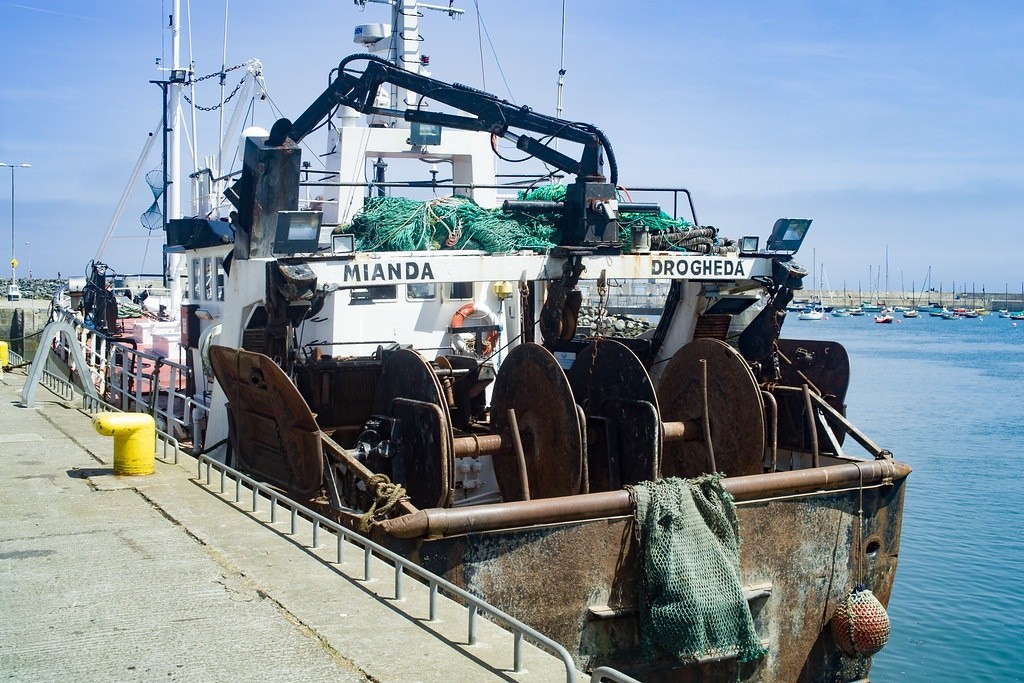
[451,302,501,358]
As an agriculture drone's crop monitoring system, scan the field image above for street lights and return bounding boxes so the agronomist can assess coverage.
[0,163,31,285]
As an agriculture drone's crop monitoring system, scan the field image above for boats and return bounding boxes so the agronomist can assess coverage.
[53,0,914,683]
[786,300,1024,323]
